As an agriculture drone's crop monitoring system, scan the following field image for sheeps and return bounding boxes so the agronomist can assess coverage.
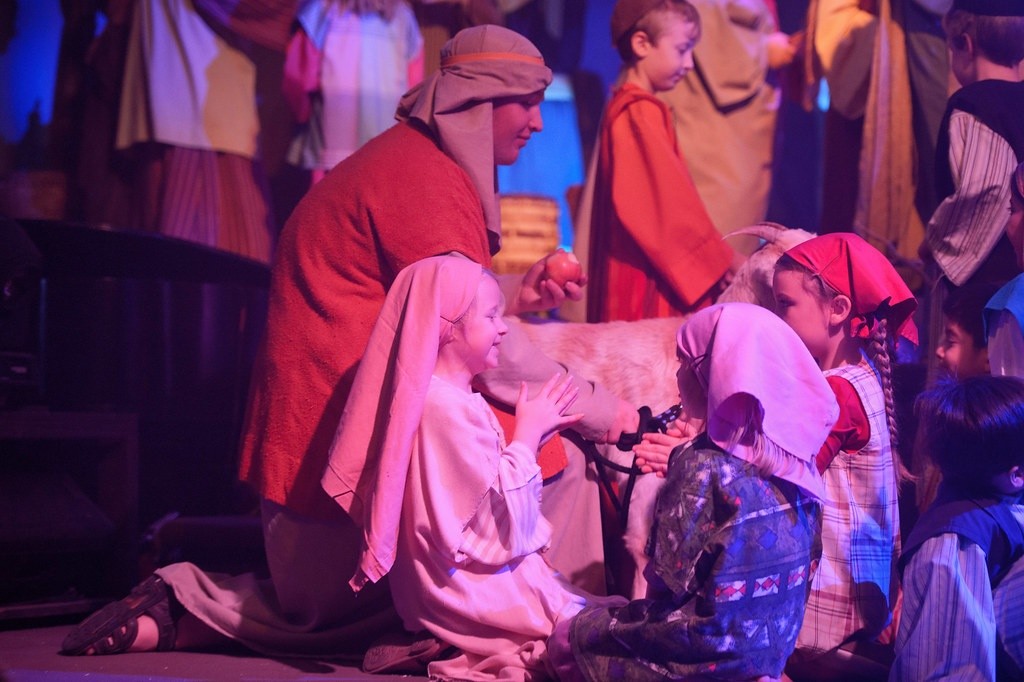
[501,219,826,617]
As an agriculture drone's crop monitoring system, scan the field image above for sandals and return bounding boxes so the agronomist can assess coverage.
[61,570,188,652]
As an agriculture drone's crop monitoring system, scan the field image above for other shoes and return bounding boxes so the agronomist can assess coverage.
[362,628,441,675]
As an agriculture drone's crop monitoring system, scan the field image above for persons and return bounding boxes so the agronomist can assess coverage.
[586,1,748,322]
[281,0,426,194]
[895,374,1024,682]
[920,0,1024,297]
[629,230,918,658]
[94,0,318,580]
[546,300,838,681]
[807,0,950,275]
[319,255,634,680]
[60,25,590,660]
[976,162,1024,387]
[558,0,808,324]
[913,276,996,386]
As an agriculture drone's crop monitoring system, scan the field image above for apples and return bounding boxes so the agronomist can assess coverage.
[545,252,583,291]
